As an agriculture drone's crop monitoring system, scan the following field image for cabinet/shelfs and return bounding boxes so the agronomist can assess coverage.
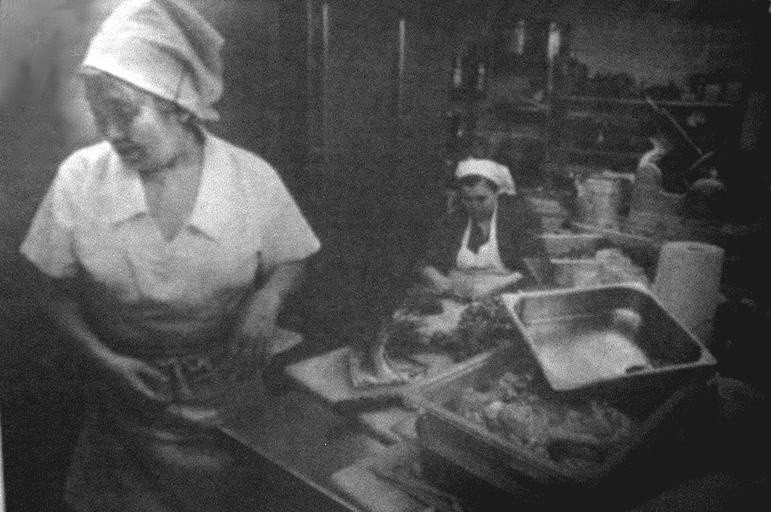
[441,18,739,185]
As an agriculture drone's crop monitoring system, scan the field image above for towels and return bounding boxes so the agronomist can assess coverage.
[332,444,463,512]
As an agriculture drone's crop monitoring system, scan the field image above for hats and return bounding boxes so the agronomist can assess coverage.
[454,155,517,196]
[81,1,232,124]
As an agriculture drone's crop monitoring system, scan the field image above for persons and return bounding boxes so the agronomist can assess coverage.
[15,1,322,512]
[408,157,555,305]
